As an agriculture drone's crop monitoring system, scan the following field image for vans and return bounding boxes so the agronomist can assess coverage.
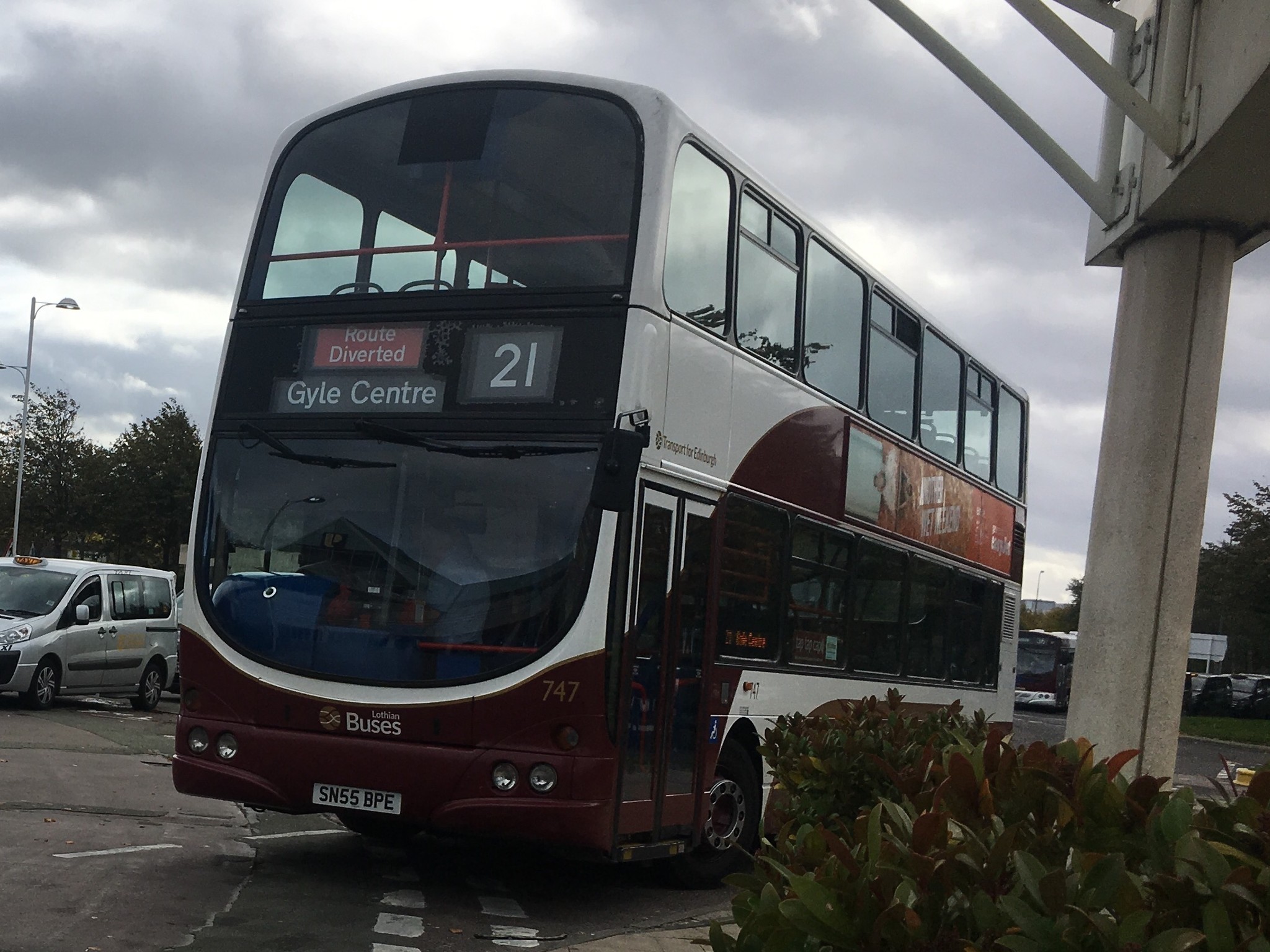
[0,556,178,711]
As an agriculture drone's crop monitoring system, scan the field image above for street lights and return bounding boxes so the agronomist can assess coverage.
[0,298,80,558]
[1035,571,1046,615]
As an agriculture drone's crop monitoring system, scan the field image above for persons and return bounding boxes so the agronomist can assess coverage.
[421,525,489,678]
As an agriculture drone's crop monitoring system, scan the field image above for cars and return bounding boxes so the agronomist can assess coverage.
[1183,672,1270,716]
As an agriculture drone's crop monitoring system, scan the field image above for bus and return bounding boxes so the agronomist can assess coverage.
[169,67,1031,884]
[1014,630,1079,713]
